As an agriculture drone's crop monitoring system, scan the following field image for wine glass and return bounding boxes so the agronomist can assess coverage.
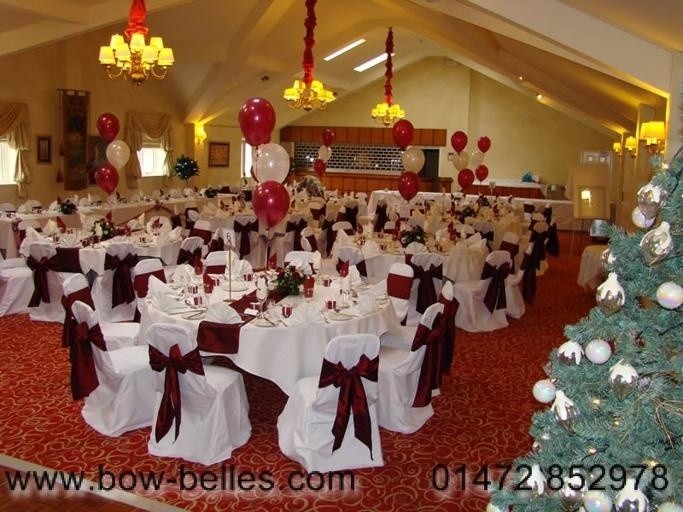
[256,286,268,319]
[95,227,102,248]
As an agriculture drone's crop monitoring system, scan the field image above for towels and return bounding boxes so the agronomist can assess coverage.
[406,241,429,254]
[335,264,363,288]
[204,301,242,323]
[146,277,172,312]
[225,260,254,279]
[172,263,195,283]
[365,239,381,253]
[58,236,85,248]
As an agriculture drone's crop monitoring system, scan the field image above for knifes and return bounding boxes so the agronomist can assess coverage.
[185,312,204,319]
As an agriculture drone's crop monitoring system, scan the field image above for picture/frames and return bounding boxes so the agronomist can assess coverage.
[35,135,52,163]
[208,142,231,167]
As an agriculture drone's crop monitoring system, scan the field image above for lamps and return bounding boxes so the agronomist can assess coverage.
[640,120,665,152]
[613,142,620,155]
[624,136,636,158]
[99,0,176,86]
[195,130,206,147]
[371,25,406,125]
[282,0,338,111]
[574,186,608,220]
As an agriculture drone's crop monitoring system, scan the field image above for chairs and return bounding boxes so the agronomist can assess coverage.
[501,194,556,243]
[270,213,308,269]
[409,253,445,314]
[387,262,413,326]
[284,250,321,274]
[498,231,519,274]
[146,322,252,466]
[176,236,204,265]
[503,241,534,320]
[234,216,269,270]
[336,246,367,277]
[19,226,46,256]
[90,242,138,322]
[454,250,514,333]
[277,333,385,475]
[209,228,225,251]
[60,273,141,362]
[132,258,166,315]
[380,279,461,370]
[288,176,367,230]
[1,183,249,224]
[454,192,500,250]
[26,242,66,324]
[205,250,239,274]
[67,300,159,439]
[0,267,35,317]
[368,188,455,231]
[0,252,26,267]
[300,225,336,274]
[378,302,450,435]
[190,220,211,247]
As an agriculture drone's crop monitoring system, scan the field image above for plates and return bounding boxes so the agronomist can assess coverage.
[327,309,352,320]
[181,311,206,320]
[249,316,278,327]
[222,279,249,291]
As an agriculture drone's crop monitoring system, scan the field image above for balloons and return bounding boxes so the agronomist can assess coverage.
[451,129,490,189]
[238,97,291,229]
[94,112,131,194]
[392,118,425,202]
[314,128,336,178]
